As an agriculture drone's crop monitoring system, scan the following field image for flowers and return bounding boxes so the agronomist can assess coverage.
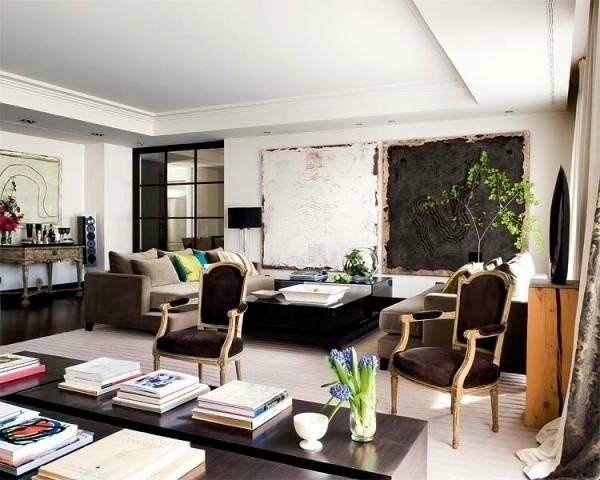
[0,175,24,232]
[319,346,378,423]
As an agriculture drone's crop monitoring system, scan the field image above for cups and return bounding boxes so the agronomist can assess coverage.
[26,222,43,244]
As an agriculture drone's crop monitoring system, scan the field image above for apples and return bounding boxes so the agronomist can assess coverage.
[335,273,349,282]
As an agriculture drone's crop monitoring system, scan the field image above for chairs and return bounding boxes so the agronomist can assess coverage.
[388,269,518,450]
[152,262,248,387]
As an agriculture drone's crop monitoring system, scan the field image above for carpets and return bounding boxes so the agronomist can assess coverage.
[0,323,542,480]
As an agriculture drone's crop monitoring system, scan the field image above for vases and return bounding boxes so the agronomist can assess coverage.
[1,231,12,245]
[349,403,378,443]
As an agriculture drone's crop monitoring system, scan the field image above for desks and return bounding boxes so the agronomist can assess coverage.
[1,243,86,308]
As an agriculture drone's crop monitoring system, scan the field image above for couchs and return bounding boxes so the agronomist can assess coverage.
[83,246,274,339]
[377,251,535,374]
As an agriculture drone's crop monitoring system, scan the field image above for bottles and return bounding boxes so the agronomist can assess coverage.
[44,224,55,242]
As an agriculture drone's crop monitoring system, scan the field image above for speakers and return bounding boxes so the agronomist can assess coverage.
[79,213,98,267]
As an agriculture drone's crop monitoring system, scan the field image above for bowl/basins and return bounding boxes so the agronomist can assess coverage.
[278,282,351,305]
[249,289,281,300]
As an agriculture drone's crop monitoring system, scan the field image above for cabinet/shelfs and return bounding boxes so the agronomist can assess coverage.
[524,279,580,430]
[274,276,392,328]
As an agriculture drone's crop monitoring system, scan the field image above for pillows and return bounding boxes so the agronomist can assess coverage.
[441,261,486,294]
[108,246,260,288]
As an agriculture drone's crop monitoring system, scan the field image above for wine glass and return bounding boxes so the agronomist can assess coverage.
[293,411,329,452]
[57,228,71,241]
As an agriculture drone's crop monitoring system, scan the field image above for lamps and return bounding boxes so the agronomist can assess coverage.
[228,206,262,256]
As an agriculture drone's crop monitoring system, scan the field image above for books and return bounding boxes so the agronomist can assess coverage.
[0,353,292,479]
[290,270,327,280]
